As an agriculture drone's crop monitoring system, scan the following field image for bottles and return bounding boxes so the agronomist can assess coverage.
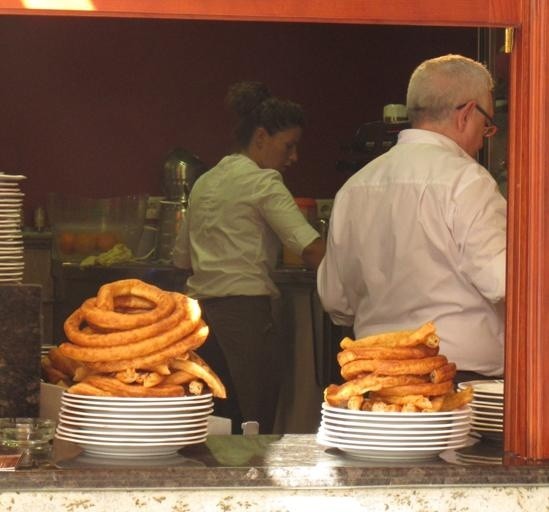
[33,208,45,233]
[280,197,316,268]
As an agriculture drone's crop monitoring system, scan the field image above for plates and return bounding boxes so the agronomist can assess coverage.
[438,442,503,467]
[52,388,214,455]
[457,380,505,433]
[55,451,204,468]
[315,402,481,461]
[0,172,30,286]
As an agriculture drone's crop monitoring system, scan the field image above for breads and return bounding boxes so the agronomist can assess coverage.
[40,277,227,400]
[322,320,476,414]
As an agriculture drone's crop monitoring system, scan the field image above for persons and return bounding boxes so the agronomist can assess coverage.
[315,51,507,396]
[185,83,326,437]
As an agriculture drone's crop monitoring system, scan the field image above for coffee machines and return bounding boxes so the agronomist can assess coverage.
[156,146,210,267]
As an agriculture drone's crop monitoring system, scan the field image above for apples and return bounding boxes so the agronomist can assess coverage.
[60,231,118,253]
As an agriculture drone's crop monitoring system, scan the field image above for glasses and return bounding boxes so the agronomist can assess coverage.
[457,103,497,137]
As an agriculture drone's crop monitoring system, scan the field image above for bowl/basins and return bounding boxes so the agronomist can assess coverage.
[48,192,149,262]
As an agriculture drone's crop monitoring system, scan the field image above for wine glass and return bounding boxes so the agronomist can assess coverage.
[0,417,56,467]
